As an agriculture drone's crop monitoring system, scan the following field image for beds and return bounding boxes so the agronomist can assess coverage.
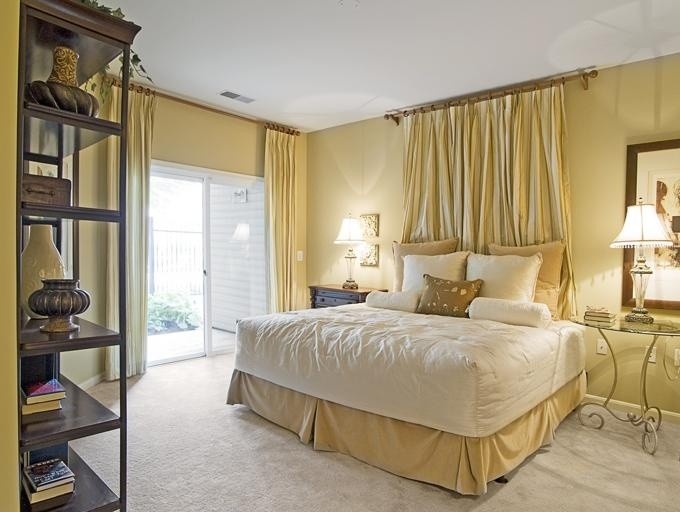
[227,302,589,497]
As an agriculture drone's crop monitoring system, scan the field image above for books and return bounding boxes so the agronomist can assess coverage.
[23,456,75,492]
[585,311,612,317]
[21,476,74,504]
[24,440,69,468]
[584,313,617,323]
[19,397,64,415]
[21,373,67,404]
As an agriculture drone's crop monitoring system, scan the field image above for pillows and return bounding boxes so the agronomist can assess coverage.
[365,237,566,328]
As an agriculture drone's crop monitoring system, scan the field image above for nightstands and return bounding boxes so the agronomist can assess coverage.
[308,283,388,309]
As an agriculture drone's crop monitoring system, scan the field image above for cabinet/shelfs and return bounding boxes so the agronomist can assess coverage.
[17,1,142,512]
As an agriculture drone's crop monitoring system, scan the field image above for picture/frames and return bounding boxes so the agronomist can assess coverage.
[621,138,680,309]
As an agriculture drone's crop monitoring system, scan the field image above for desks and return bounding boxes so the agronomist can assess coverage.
[568,306,680,454]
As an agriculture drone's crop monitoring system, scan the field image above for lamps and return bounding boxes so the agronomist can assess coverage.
[611,203,674,325]
[333,217,367,289]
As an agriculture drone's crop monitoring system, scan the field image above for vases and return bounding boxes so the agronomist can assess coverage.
[19,224,64,319]
